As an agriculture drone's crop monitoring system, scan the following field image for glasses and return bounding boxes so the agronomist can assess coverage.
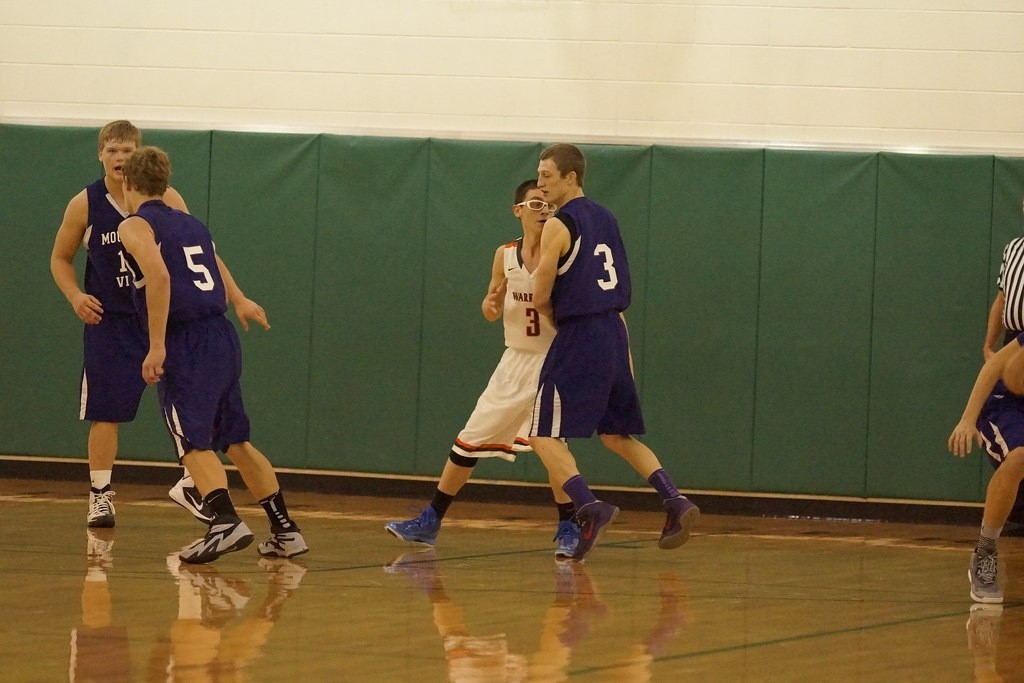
[517,201,559,214]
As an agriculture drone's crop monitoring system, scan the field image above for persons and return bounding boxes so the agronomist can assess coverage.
[530,143,701,557]
[50,121,271,525]
[945,235,1024,603]
[965,604,1004,683]
[383,179,581,557]
[67,527,201,683]
[176,558,309,682]
[383,554,690,683]
[117,146,309,561]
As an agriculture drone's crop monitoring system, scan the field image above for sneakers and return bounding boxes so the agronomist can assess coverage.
[86,485,116,527]
[966,548,1003,605]
[179,515,254,565]
[179,564,250,610]
[257,527,308,557]
[86,527,116,573]
[384,548,438,586]
[571,495,622,562]
[658,496,699,549]
[386,506,441,544]
[553,520,581,557]
[169,474,216,524]
[258,557,308,590]
[966,600,1002,652]
[552,564,608,625]
